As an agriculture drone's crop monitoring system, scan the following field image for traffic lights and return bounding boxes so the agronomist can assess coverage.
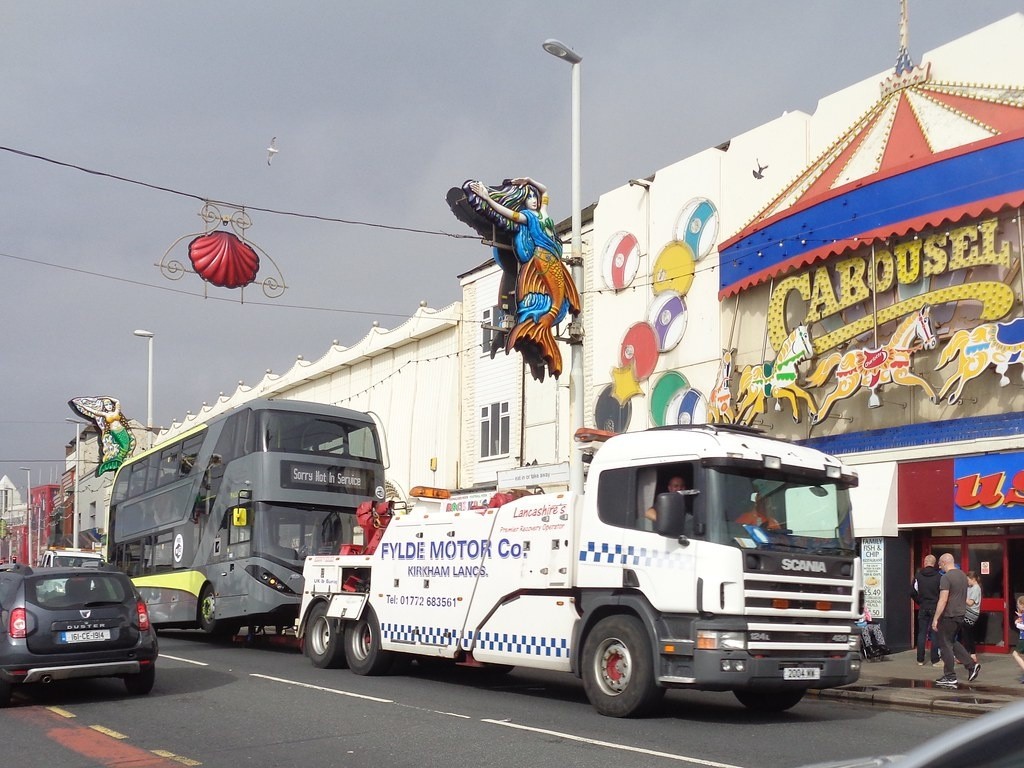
[13,557,16,563]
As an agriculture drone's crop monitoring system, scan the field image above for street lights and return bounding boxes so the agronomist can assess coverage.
[65,417,81,548]
[19,466,33,566]
[541,37,585,488]
[133,329,154,429]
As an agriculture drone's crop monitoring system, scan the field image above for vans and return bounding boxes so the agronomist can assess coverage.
[35,547,105,593]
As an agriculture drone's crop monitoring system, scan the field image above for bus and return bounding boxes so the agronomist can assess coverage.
[106,394,386,639]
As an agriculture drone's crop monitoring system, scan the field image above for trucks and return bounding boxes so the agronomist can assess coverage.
[295,422,868,720]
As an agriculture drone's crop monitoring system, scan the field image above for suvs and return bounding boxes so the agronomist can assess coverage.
[0,564,160,708]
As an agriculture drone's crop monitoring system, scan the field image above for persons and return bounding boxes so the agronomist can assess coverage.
[645,477,692,521]
[738,493,780,529]
[932,554,981,684]
[910,555,943,666]
[956,572,981,664]
[861,606,891,654]
[1012,596,1024,683]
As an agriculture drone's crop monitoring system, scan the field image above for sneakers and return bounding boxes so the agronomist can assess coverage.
[968,663,980,682]
[936,674,958,684]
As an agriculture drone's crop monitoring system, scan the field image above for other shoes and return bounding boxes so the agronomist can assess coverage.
[917,661,924,666]
[1020,675,1024,684]
[879,645,890,655]
[956,658,976,663]
[865,646,881,658]
[933,660,943,667]
